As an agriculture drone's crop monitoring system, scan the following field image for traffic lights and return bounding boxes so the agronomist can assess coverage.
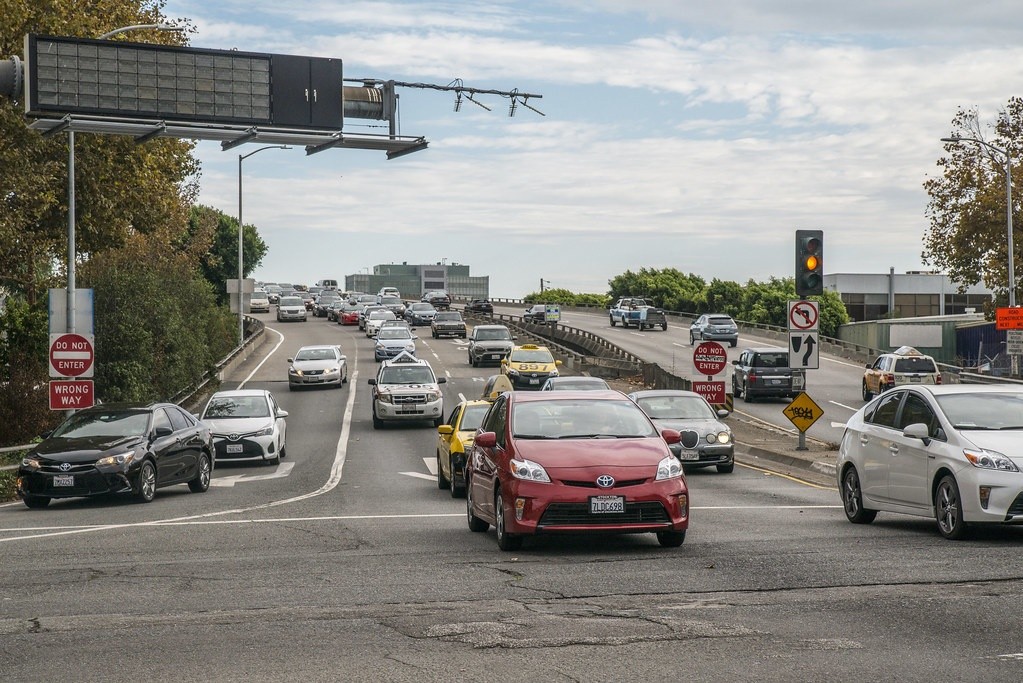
[795,230,825,297]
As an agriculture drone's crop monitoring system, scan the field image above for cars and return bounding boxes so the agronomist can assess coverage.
[251,278,466,361]
[690,313,740,346]
[288,344,348,390]
[837,382,1023,539]
[19,402,216,509]
[193,389,289,466]
[436,374,736,553]
[500,345,560,389]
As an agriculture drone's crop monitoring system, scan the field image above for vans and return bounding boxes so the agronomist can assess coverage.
[608,297,668,330]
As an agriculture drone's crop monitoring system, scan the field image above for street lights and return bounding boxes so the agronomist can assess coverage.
[940,137,1019,376]
[239,143,294,345]
[540,279,551,291]
[62,24,185,420]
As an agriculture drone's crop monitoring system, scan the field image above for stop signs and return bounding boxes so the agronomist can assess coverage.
[694,341,726,374]
[47,328,95,378]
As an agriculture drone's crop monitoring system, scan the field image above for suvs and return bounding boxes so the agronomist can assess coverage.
[465,299,494,317]
[862,345,944,402]
[469,325,520,367]
[732,350,807,403]
[524,303,558,325]
[368,352,448,427]
